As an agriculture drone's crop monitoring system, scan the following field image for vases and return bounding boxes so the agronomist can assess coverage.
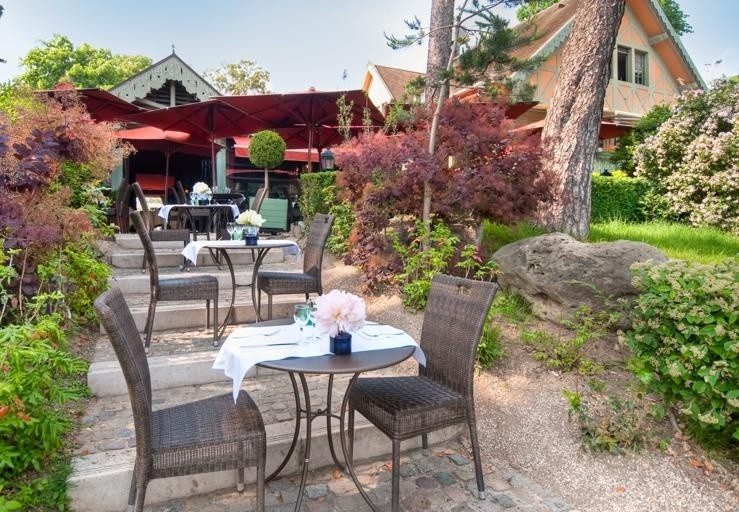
[329,331,351,355]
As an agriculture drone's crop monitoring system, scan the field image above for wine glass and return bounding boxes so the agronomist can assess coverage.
[223,187,233,205]
[307,298,324,340]
[226,222,236,243]
[234,221,244,243]
[190,186,220,206]
[293,305,310,341]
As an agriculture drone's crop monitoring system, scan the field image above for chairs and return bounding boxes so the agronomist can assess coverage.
[130,181,190,273]
[257,212,337,320]
[345,273,499,512]
[168,181,268,242]
[92,286,266,512]
[129,210,219,354]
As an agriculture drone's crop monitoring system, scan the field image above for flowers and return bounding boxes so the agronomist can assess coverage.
[311,289,368,338]
